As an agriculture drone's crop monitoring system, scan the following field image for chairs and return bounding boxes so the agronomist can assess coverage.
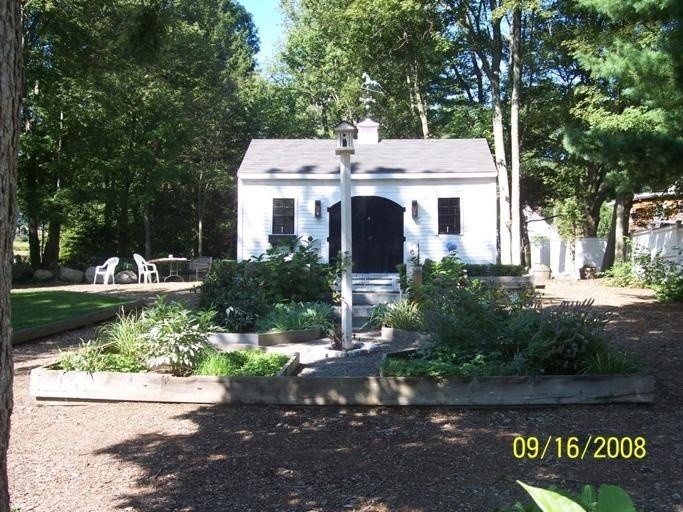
[188,255,213,281]
[92,256,119,284]
[133,253,160,282]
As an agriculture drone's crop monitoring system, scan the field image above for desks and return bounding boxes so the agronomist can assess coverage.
[149,256,189,283]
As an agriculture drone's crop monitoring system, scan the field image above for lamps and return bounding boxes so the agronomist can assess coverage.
[411,200,417,217]
[314,200,320,217]
